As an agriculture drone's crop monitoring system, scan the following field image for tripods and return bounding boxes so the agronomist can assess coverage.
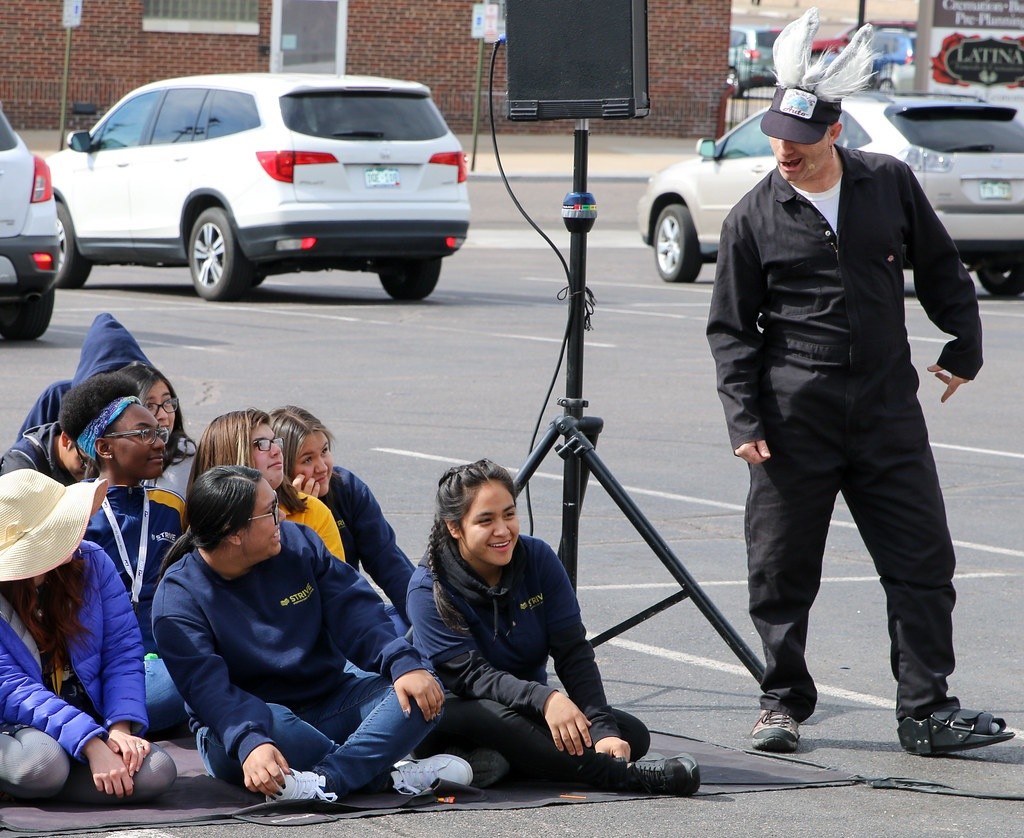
[508,119,771,692]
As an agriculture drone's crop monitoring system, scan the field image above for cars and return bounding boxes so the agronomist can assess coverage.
[809,18,917,99]
[725,25,785,100]
[0,112,65,342]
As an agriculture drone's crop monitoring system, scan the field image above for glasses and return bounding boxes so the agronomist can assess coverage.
[248,490,279,526]
[252,437,285,451]
[101,427,169,445]
[75,442,91,470]
[143,398,179,416]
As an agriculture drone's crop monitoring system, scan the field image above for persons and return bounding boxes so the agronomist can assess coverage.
[151,466,473,802]
[270,406,418,636]
[706,86,1016,753]
[60,372,190,732]
[181,407,345,561]
[120,362,197,499]
[404,460,701,796]
[16,313,152,443]
[0,421,90,487]
[0,469,177,804]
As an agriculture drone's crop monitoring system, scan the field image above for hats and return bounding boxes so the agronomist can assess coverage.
[0,468,109,581]
[760,6,883,145]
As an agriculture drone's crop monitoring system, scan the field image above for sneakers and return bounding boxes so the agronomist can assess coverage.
[750,709,799,751]
[626,752,700,797]
[390,753,473,795]
[266,763,338,802]
[444,747,510,788]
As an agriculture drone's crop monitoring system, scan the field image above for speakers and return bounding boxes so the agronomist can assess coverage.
[505,0,650,122]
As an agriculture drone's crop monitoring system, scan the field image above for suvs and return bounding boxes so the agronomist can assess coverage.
[40,72,472,303]
[636,90,1024,298]
